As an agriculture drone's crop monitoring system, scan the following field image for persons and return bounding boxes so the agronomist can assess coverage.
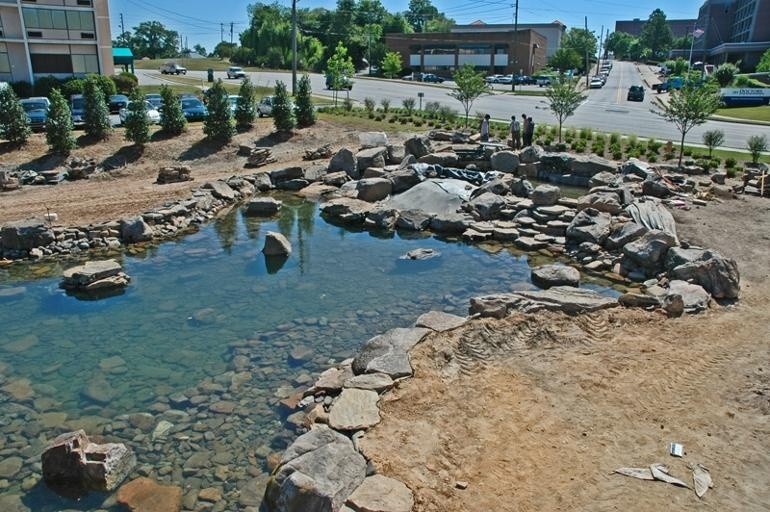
[480,114,490,139]
[510,116,521,150]
[522,113,528,148]
[526,116,534,145]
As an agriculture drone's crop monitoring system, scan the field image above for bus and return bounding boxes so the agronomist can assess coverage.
[709,72,770,109]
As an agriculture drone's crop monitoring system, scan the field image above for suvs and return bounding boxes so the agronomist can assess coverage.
[627,85,646,102]
[226,66,246,80]
[652,77,693,94]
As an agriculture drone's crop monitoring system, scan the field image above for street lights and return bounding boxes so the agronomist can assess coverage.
[684,22,705,97]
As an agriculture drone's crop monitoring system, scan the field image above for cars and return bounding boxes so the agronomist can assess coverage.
[590,60,613,88]
[485,67,578,88]
[257,94,296,117]
[400,70,445,83]
[229,94,258,118]
[324,74,353,91]
[18,93,209,133]
[660,60,718,90]
[159,61,188,76]
